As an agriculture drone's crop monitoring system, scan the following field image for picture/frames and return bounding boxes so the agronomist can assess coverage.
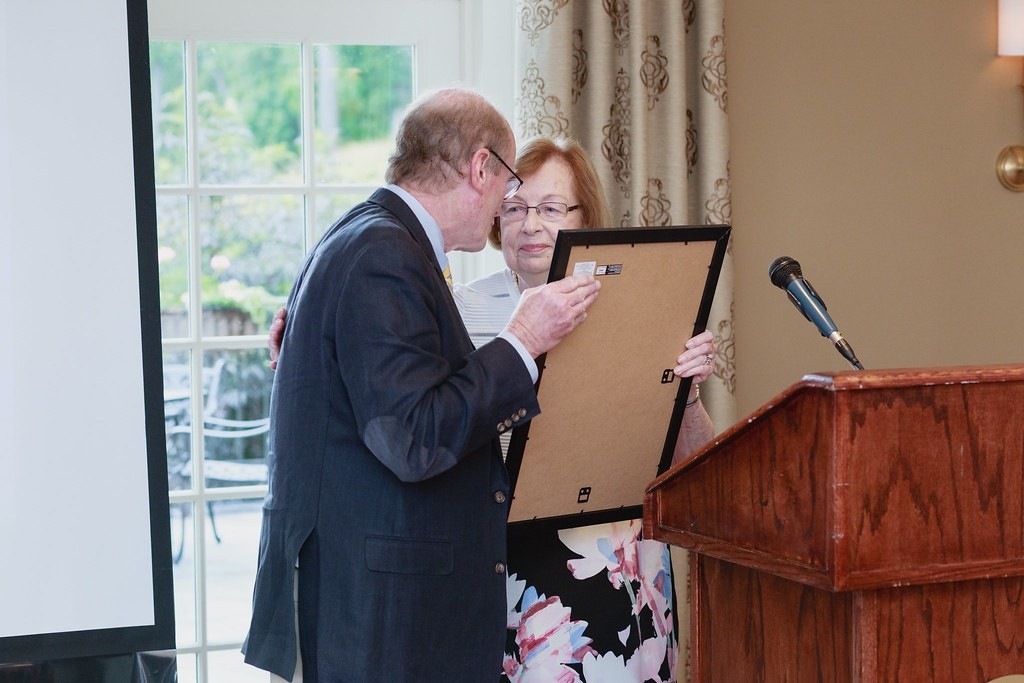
[504,224,733,538]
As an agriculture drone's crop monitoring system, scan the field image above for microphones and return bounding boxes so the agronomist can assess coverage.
[767,255,859,365]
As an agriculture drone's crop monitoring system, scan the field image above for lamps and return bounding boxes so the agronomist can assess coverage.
[995,0,1024,195]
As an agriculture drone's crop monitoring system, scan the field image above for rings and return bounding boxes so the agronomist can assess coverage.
[704,354,713,364]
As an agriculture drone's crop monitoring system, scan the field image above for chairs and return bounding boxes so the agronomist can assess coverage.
[164,357,269,563]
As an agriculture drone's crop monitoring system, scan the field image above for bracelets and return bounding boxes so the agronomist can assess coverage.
[686,385,699,407]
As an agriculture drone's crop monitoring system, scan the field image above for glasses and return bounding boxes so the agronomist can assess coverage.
[485,147,523,200]
[499,202,582,223]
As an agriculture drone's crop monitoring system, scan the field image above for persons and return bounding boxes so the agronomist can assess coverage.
[241,89,718,683]
